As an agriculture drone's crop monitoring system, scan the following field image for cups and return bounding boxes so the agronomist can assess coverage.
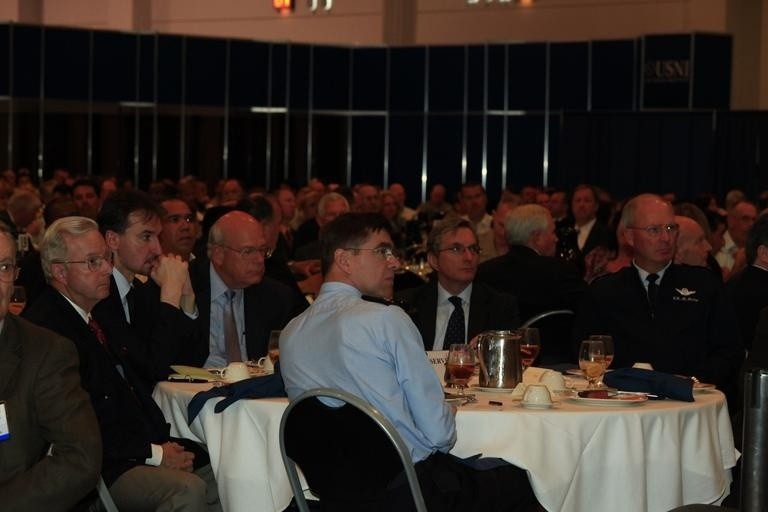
[219,363,251,384]
[258,357,273,376]
[537,371,569,388]
[524,384,558,403]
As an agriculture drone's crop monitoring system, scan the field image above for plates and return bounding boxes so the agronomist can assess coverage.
[693,381,717,394]
[568,391,650,406]
[476,386,524,395]
[520,402,556,410]
[552,386,572,396]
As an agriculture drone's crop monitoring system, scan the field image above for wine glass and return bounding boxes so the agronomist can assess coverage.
[9,284,27,319]
[561,242,575,263]
[446,345,476,406]
[519,325,540,368]
[579,341,606,389]
[588,336,615,389]
[398,251,410,278]
[269,327,282,361]
[412,252,429,277]
[8,286,27,315]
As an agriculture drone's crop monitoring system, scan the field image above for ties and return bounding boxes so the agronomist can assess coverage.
[442,297,465,351]
[222,291,243,366]
[645,273,660,311]
[87,317,109,344]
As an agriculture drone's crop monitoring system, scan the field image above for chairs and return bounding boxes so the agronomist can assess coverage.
[276,386,432,511]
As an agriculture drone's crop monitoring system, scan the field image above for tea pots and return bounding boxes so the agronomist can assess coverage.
[477,309,573,388]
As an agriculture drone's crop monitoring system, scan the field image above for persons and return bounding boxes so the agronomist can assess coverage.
[185,214,298,390]
[404,217,513,352]
[26,193,207,506]
[1,232,103,511]
[722,215,768,394]
[587,195,722,388]
[558,185,614,273]
[275,213,544,511]
[699,184,748,218]
[718,202,759,271]
[476,204,573,333]
[672,216,716,270]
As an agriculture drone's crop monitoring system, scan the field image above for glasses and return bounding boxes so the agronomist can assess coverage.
[625,225,677,236]
[0,264,20,282]
[165,215,196,227]
[50,252,112,272]
[215,243,272,259]
[438,245,480,256]
[341,247,398,260]
